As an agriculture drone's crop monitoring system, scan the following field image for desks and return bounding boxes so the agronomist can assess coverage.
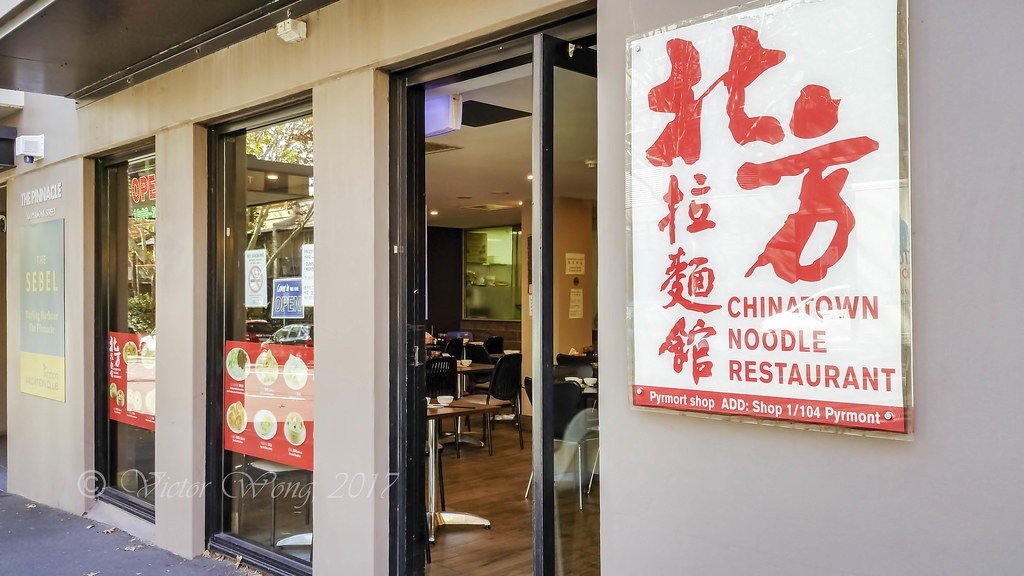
[439,363,495,448]
[426,399,501,543]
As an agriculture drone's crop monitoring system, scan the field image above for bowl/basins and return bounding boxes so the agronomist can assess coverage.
[461,360,472,366]
[583,377,597,386]
[565,377,582,384]
[437,396,454,406]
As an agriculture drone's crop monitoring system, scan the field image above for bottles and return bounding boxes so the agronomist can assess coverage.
[429,333,446,345]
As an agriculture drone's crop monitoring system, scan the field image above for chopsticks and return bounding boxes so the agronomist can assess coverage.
[443,405,476,409]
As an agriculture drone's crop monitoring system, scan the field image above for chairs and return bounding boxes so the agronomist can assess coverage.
[523,354,600,510]
[426,336,524,459]
[237,453,312,546]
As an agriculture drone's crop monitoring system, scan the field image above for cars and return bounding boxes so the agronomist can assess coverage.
[246,319,275,343]
[258,323,314,368]
[139,327,156,369]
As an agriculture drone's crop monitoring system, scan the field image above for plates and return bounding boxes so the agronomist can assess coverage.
[253,410,277,440]
[284,420,306,446]
[427,405,444,409]
[255,352,278,386]
[227,403,248,434]
[282,357,308,391]
[225,348,251,382]
[123,341,155,370]
